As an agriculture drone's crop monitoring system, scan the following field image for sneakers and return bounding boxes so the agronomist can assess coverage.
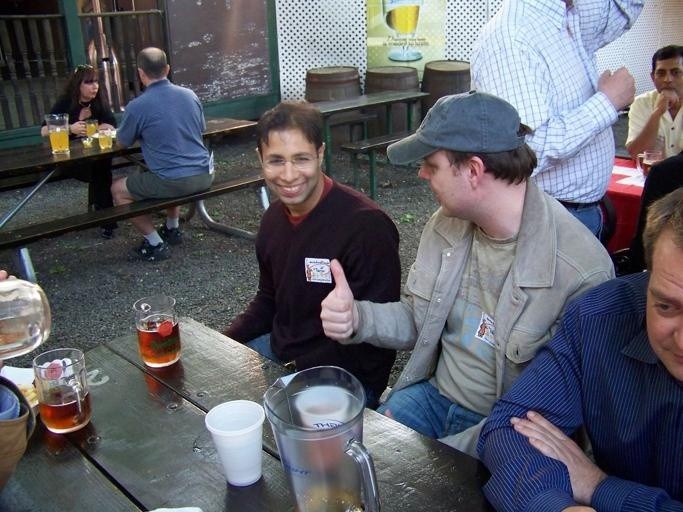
[159,224,181,244]
[128,239,171,260]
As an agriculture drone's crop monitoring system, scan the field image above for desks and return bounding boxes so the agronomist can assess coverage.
[597,156,668,256]
[0,117,260,240]
[310,88,429,176]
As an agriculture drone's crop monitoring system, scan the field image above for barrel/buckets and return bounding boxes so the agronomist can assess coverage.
[364,66,423,151]
[420,60,471,121]
[305,66,365,152]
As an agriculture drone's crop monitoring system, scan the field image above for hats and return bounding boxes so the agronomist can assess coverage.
[387,91,525,166]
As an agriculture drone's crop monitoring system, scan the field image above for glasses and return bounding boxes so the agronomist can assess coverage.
[74,64,93,73]
[262,153,319,169]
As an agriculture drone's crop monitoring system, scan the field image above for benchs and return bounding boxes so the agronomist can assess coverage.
[321,112,377,156]
[1,171,271,283]
[341,127,419,200]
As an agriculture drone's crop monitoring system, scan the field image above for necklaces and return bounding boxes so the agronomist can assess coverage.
[80,102,91,112]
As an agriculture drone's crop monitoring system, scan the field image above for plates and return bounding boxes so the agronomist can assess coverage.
[0,358,88,415]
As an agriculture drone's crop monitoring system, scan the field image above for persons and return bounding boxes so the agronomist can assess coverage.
[41,64,118,239]
[117,47,215,261]
[477,188,682,512]
[221,103,400,410]
[319,91,615,463]
[469,0,643,241]
[624,45,681,174]
[0,270,37,494]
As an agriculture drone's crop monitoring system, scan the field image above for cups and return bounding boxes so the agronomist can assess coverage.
[132,294,182,367]
[204,364,380,510]
[31,347,91,435]
[81,119,112,149]
[636,150,662,184]
[0,278,51,360]
[42,113,71,156]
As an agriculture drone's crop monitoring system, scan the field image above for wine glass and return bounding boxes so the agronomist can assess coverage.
[382,0,423,62]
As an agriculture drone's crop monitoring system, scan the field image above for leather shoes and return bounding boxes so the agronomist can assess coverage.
[97,225,112,239]
